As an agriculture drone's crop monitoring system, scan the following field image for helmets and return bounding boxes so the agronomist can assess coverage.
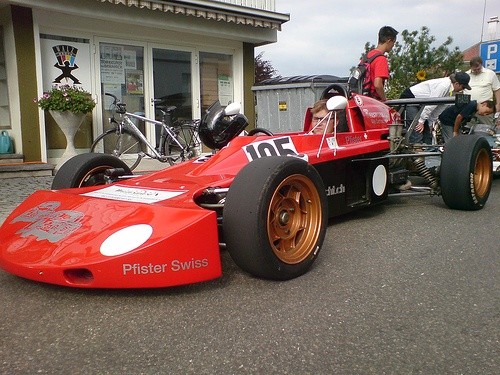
[198,100,249,151]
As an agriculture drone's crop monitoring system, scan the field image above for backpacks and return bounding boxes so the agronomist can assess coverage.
[345,48,387,100]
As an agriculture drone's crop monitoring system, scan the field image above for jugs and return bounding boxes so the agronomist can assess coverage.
[0,130,14,154]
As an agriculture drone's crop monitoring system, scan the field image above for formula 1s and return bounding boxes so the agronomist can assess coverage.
[1,84,496,288]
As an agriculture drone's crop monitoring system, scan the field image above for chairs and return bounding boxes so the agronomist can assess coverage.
[322,85,348,135]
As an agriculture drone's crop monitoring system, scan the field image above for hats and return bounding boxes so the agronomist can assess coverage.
[454,72,472,90]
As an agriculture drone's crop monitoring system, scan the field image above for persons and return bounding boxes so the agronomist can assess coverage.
[309,99,344,135]
[397,57,500,152]
[358,25,399,101]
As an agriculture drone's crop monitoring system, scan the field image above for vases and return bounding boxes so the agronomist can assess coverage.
[47,107,88,176]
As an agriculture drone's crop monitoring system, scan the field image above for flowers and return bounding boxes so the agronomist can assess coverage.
[32,83,97,115]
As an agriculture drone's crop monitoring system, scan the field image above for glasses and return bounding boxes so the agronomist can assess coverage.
[312,116,334,121]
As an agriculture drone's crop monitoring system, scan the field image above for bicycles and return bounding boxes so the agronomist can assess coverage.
[89,92,217,167]
